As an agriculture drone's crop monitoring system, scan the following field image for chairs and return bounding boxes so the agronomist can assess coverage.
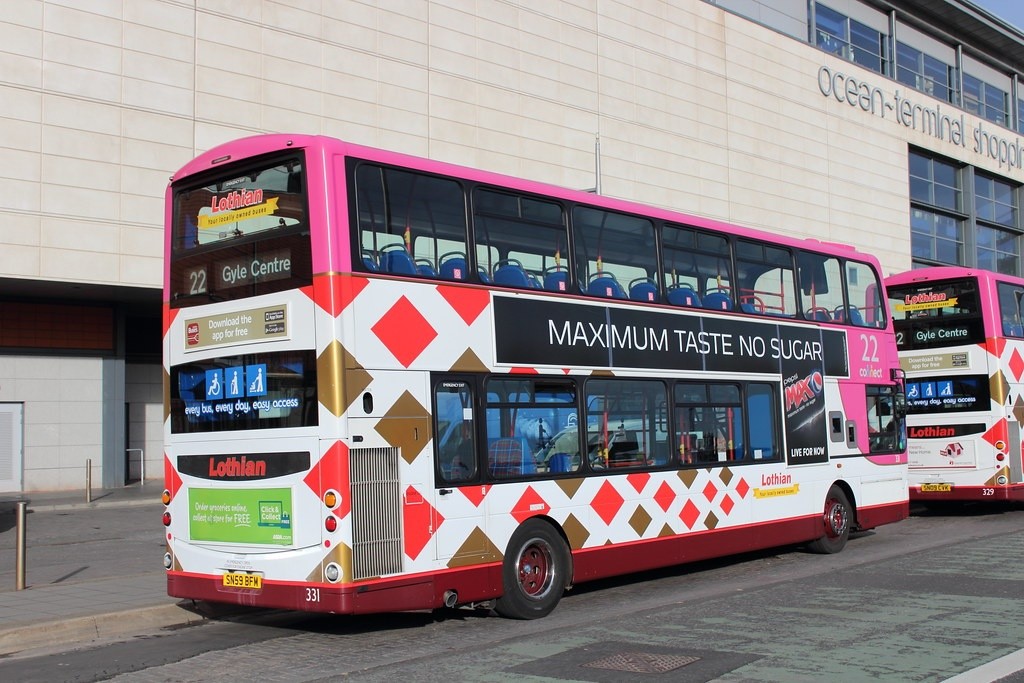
[358,241,867,327]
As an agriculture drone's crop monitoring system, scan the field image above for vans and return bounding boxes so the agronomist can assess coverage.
[532,417,706,473]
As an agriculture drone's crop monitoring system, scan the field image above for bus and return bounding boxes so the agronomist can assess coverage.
[160,131,913,621]
[864,266,1023,504]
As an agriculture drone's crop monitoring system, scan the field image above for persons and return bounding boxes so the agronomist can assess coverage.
[1002,297,1021,336]
